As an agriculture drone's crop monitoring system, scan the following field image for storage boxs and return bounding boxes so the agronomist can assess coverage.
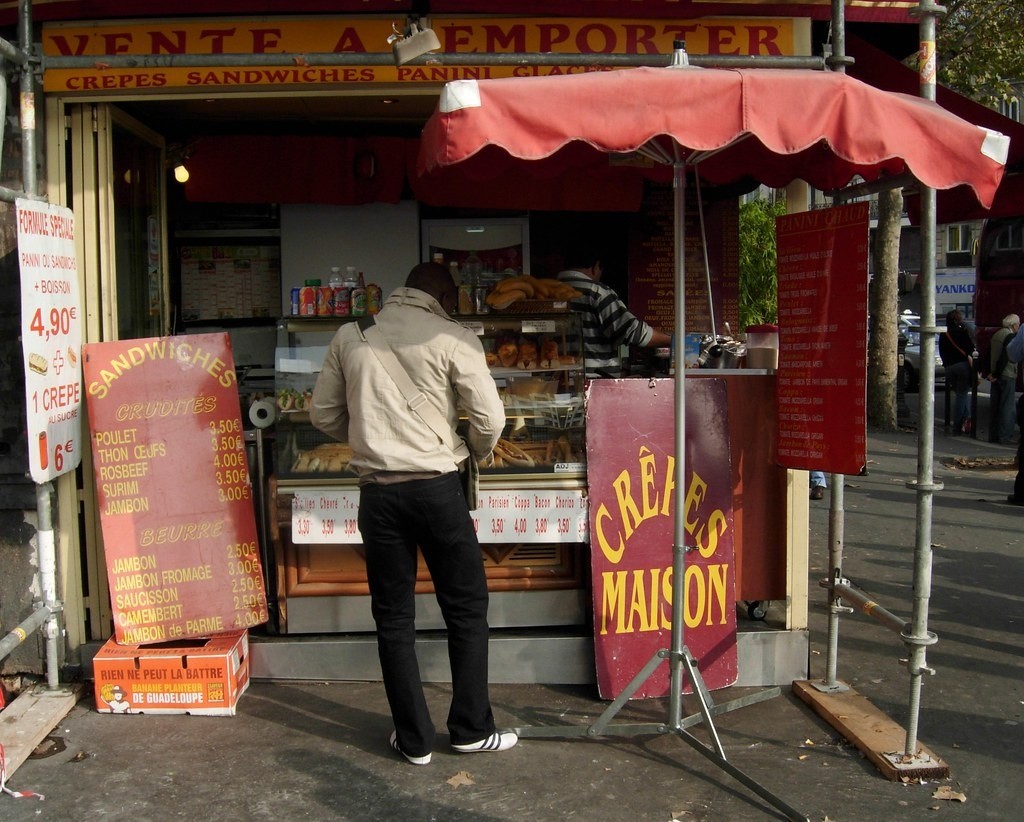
[91,627,253,717]
[271,308,589,485]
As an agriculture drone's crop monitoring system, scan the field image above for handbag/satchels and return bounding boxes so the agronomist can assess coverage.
[457,436,481,510]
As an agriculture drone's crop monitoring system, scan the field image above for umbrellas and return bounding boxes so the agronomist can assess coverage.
[417,39,1012,822]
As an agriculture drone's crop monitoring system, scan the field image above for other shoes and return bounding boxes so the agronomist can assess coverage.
[1007,494,1024,506]
[453,734,519,752]
[810,486,825,500]
[390,728,433,766]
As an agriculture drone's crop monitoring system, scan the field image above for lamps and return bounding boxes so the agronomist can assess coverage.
[387,23,443,66]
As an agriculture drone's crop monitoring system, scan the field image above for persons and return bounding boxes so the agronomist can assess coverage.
[1007,322,1024,507]
[982,314,1020,444]
[543,244,671,387]
[307,261,520,765]
[939,310,976,437]
[809,470,827,499]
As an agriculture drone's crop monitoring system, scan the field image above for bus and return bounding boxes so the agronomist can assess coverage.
[972,215,1024,427]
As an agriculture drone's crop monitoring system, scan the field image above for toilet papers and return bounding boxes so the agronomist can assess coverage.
[249,393,278,428]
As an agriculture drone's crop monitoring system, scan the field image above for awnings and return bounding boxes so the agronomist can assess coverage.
[812,33,1024,226]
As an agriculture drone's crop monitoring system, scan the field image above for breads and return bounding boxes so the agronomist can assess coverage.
[277,388,351,473]
[477,274,584,469]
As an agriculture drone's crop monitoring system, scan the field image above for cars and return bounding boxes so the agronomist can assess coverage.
[896,309,947,391]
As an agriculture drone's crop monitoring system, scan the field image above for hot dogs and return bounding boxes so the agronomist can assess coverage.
[66,347,78,368]
[28,353,49,376]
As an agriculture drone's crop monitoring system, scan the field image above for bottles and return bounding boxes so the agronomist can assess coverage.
[343,266,360,289]
[448,261,463,286]
[459,250,483,287]
[329,266,345,289]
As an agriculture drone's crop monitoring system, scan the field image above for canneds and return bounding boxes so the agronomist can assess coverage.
[290,276,382,316]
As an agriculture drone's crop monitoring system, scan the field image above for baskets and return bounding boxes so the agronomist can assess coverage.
[491,297,570,314]
